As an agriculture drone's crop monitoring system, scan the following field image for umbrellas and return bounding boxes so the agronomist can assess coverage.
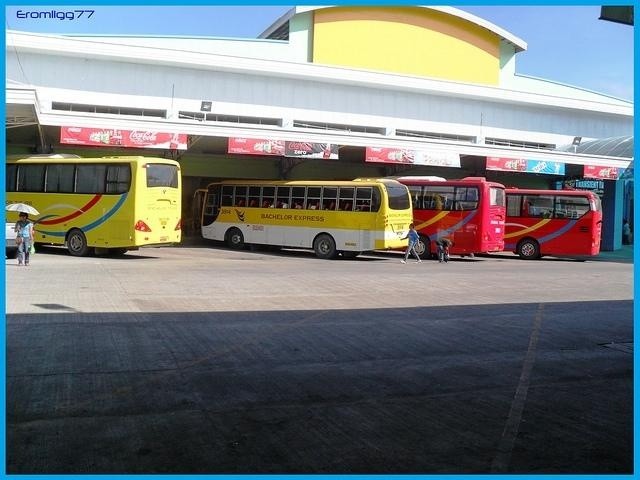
[5,202,40,231]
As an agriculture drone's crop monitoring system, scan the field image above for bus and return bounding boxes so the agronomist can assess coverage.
[501,187,604,262]
[191,175,416,261]
[6,152,183,257]
[389,173,507,260]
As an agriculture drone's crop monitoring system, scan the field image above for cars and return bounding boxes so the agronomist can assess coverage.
[5,221,18,259]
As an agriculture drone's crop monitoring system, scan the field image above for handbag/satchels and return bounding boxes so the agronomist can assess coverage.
[16,236,24,245]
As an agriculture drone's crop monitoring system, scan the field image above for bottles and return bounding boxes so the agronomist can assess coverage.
[170,133,179,150]
[102,129,123,144]
[324,143,331,159]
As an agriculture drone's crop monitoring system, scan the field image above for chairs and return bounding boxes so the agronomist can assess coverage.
[238,200,367,211]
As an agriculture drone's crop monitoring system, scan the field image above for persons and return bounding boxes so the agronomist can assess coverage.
[12,212,35,266]
[435,236,454,264]
[623,218,632,245]
[400,223,423,264]
